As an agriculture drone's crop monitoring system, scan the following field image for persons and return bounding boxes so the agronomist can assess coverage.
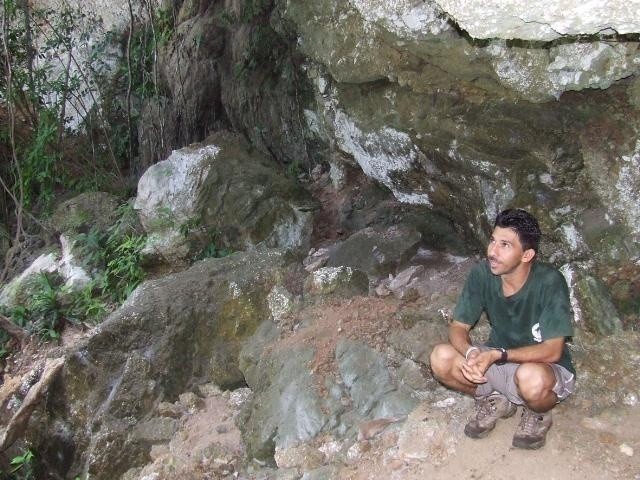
[428,207,578,451]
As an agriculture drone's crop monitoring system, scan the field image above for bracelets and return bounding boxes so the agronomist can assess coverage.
[465,347,478,360]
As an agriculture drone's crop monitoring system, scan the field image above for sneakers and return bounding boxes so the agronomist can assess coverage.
[464,395,516,438]
[512,405,551,450]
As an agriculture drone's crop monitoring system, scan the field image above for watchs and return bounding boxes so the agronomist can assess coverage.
[493,347,508,367]
